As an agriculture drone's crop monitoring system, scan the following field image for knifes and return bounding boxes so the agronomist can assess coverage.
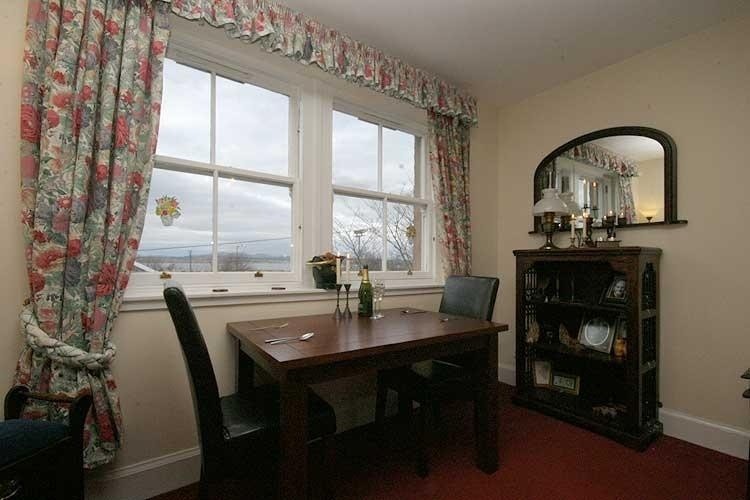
[265,336,294,343]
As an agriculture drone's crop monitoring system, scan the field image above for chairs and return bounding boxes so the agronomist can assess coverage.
[374,275,500,478]
[164,287,337,500]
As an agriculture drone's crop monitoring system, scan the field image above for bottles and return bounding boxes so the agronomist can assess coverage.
[358,264,373,318]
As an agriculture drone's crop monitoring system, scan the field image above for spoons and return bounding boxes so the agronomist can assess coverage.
[401,309,426,314]
[270,332,315,345]
[249,322,291,332]
[439,316,466,323]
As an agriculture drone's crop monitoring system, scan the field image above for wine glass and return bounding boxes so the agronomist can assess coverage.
[369,283,385,320]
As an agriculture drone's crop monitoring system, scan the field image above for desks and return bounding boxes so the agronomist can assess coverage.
[225,306,508,500]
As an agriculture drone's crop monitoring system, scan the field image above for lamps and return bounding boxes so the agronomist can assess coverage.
[558,192,582,228]
[639,208,658,222]
[531,189,568,250]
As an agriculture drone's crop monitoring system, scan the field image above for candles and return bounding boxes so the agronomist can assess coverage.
[570,212,587,238]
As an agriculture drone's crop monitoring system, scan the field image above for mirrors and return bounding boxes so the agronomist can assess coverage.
[528,126,688,234]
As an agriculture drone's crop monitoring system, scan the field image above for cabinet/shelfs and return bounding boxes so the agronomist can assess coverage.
[512,246,663,452]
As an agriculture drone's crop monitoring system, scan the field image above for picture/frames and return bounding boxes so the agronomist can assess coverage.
[531,359,580,396]
[599,273,629,307]
